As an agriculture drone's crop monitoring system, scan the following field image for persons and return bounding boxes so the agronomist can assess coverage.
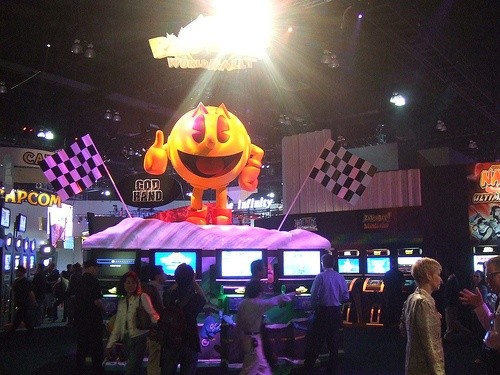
[381,256,500,375]
[105,263,160,375]
[471,205,500,241]
[159,262,206,375]
[141,265,166,375]
[74,260,111,375]
[246,260,281,375]
[50,212,66,247]
[266,258,280,296]
[112,204,120,217]
[301,254,350,374]
[239,280,302,375]
[7,258,85,338]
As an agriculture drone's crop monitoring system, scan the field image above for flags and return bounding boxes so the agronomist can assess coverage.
[308,138,378,206]
[37,134,107,202]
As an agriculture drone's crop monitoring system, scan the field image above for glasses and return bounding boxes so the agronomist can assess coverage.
[485,272,500,278]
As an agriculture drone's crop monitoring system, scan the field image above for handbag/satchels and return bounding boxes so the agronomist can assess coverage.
[117,332,132,363]
[136,294,152,330]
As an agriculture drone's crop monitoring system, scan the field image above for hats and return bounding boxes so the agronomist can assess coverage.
[84,260,101,269]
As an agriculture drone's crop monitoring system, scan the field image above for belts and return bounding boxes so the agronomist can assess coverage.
[245,331,262,335]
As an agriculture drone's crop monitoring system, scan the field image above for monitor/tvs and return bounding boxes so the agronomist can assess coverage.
[277,248,326,281]
[14,254,20,270]
[336,256,362,275]
[4,253,11,270]
[1,207,11,229]
[31,241,35,249]
[22,255,27,268]
[7,237,12,245]
[17,240,21,248]
[396,257,421,276]
[215,248,269,280]
[24,241,28,249]
[92,248,141,282]
[41,245,52,253]
[30,255,35,269]
[147,249,202,281]
[364,255,392,276]
[18,213,27,233]
[42,258,49,266]
